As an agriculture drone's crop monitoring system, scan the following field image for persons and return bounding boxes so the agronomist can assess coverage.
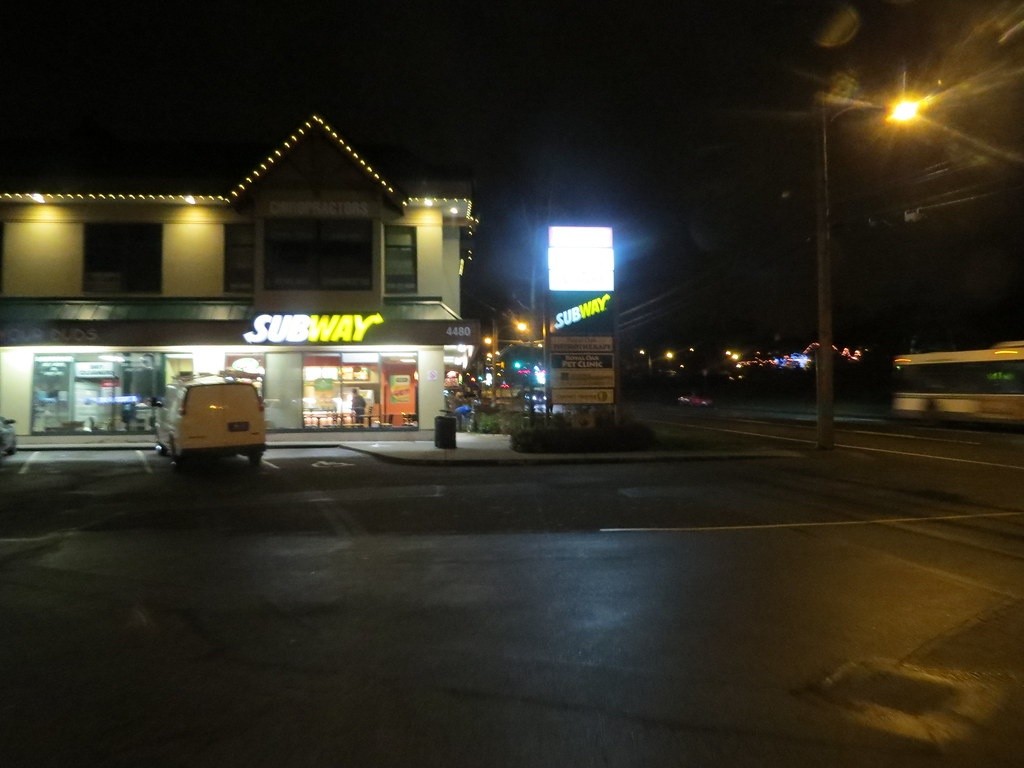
[351,389,366,428]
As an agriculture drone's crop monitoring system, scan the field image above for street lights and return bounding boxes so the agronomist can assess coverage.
[639,350,653,376]
[814,99,923,450]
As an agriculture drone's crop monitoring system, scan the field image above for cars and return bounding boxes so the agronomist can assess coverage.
[678,390,712,410]
[0,415,17,458]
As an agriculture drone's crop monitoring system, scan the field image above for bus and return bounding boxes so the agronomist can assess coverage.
[885,340,1024,426]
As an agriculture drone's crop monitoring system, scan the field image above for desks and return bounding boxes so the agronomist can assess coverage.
[359,414,376,426]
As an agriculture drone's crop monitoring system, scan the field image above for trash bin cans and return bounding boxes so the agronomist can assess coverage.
[435,416,456,448]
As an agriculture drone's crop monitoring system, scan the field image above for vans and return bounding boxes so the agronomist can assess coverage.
[149,377,267,467]
